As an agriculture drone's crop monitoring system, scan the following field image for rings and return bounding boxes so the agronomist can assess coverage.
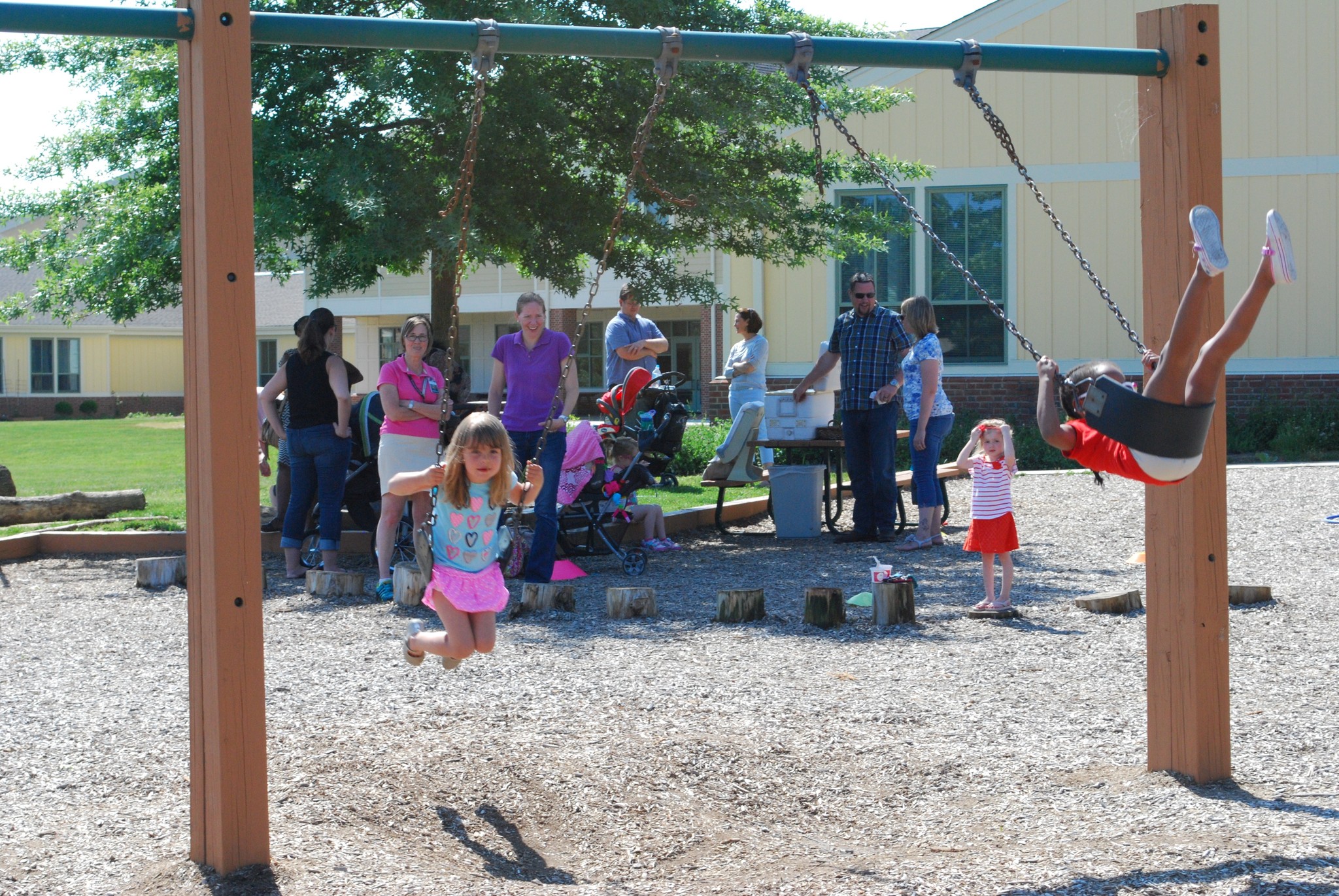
[918,444,921,446]
[635,347,637,349]
[884,397,888,398]
[550,431,553,433]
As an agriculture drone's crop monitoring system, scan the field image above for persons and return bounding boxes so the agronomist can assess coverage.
[262,315,364,535]
[1036,204,1296,486]
[604,284,668,392]
[374,316,455,601]
[599,436,682,551]
[387,410,545,671]
[487,292,578,583]
[792,271,914,544]
[259,308,351,578]
[957,419,1020,611]
[894,296,956,549]
[723,308,774,487]
[257,387,285,476]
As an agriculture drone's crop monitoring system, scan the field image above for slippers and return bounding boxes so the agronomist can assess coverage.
[288,565,318,580]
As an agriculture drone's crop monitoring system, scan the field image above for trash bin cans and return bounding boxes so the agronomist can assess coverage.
[767,464,827,539]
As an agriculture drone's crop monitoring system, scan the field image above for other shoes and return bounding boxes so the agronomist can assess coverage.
[834,531,878,542]
[404,619,425,666]
[879,529,896,543]
[260,520,281,534]
[443,654,461,670]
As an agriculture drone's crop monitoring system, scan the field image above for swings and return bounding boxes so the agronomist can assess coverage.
[413,59,676,588]
[801,76,1217,460]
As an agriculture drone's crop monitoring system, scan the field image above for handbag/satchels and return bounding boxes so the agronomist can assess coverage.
[260,412,282,448]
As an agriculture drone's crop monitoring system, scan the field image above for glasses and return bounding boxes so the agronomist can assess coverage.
[747,308,752,319]
[850,289,875,298]
[405,334,427,342]
[900,315,905,321]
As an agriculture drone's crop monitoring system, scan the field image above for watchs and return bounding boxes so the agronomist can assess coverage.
[889,379,900,389]
[558,415,569,423]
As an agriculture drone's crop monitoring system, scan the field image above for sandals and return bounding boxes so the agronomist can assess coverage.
[985,597,1012,611]
[931,534,943,545]
[894,534,932,551]
[973,597,996,611]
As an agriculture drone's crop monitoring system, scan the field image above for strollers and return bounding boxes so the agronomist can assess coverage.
[553,409,673,577]
[589,366,704,489]
[298,390,425,568]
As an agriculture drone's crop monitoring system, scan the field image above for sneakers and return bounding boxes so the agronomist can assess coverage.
[1261,209,1297,284]
[1189,205,1228,278]
[641,538,668,552]
[375,581,395,602]
[656,537,681,550]
